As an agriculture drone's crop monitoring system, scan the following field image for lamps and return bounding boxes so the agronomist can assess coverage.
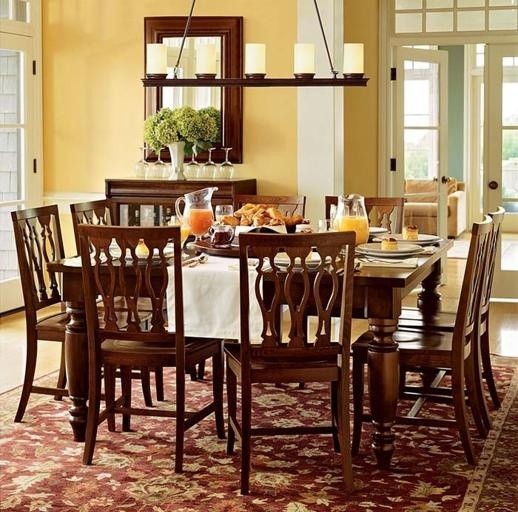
[140,1,370,87]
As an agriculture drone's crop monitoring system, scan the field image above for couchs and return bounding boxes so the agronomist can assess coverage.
[403,178,465,237]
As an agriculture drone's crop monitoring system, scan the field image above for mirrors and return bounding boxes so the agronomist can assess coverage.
[143,16,243,163]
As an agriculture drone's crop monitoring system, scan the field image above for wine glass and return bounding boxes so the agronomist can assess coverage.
[171,216,191,256]
[133,146,235,181]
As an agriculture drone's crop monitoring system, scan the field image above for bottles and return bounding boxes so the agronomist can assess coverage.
[129,205,172,226]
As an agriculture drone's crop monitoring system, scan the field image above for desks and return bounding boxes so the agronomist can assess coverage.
[105,179,257,226]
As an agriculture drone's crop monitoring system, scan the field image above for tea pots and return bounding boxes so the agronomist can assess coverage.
[173,185,219,247]
[329,192,370,248]
[209,224,235,248]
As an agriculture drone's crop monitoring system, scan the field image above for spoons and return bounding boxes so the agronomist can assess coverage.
[183,256,209,268]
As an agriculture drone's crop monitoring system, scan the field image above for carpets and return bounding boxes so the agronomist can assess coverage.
[0,353,517,512]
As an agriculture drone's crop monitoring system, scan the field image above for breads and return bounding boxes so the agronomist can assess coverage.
[222,203,303,225]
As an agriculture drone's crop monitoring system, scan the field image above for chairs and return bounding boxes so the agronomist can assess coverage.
[70,199,196,401]
[10,204,152,432]
[351,214,493,464]
[325,195,404,234]
[233,195,306,220]
[223,230,356,494]
[77,224,224,473]
[397,205,506,428]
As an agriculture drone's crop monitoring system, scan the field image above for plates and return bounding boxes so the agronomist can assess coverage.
[103,238,178,260]
[230,222,445,270]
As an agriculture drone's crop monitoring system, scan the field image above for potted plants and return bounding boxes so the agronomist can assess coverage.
[143,106,221,179]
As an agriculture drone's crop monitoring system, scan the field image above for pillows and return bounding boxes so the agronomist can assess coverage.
[405,179,457,203]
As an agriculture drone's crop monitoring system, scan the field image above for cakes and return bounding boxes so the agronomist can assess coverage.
[402,225,419,240]
[380,238,399,250]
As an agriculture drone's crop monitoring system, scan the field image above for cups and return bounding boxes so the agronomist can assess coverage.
[317,219,329,234]
[284,215,297,233]
[215,204,233,221]
